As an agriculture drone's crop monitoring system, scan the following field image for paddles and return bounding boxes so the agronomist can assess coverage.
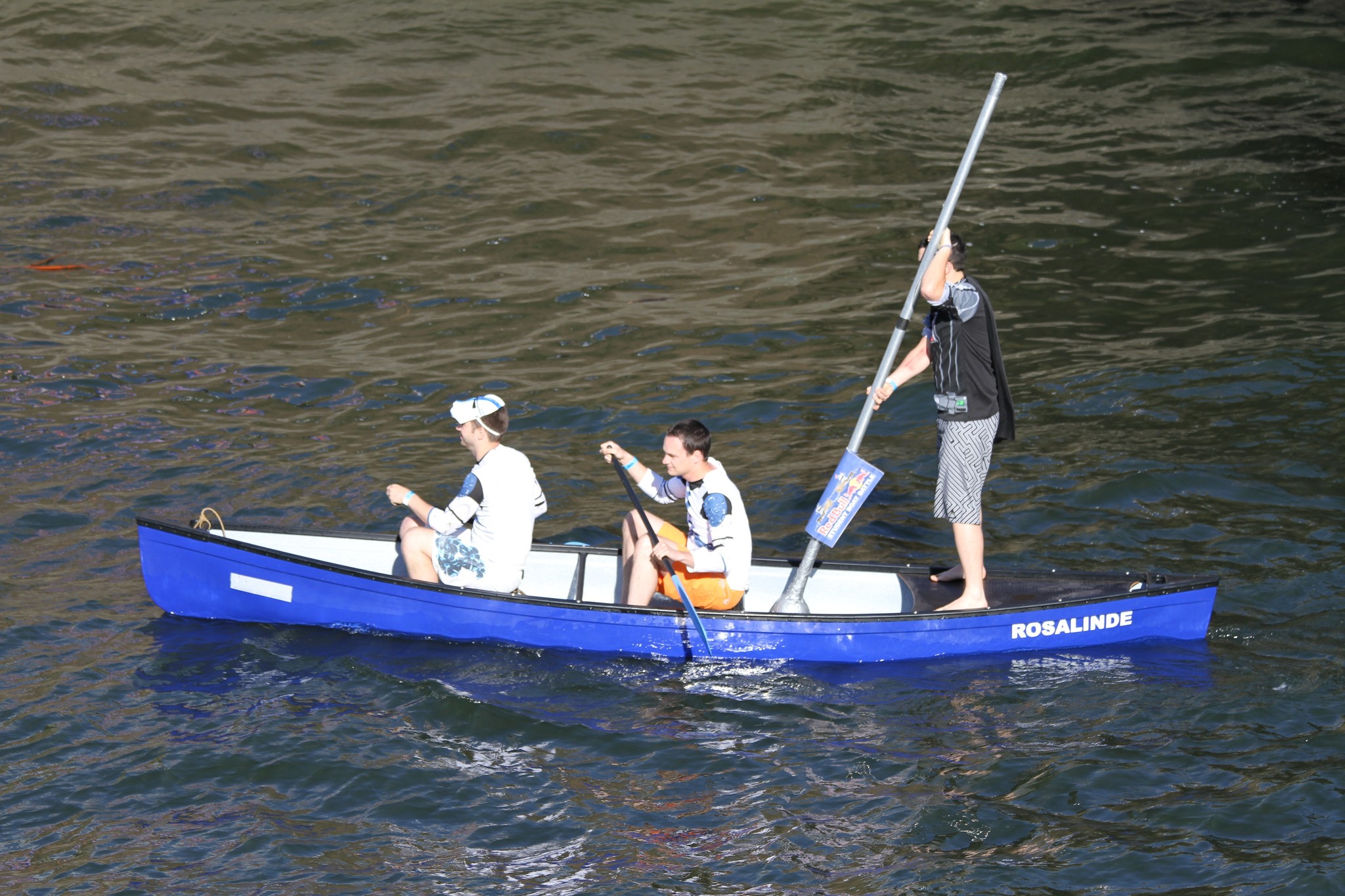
[607,446,713,656]
[467,517,591,547]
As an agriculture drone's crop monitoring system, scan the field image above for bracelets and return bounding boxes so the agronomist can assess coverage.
[885,379,897,393]
[622,457,639,472]
[402,490,416,507]
[936,243,953,253]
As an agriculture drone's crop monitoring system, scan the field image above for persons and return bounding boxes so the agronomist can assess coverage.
[385,393,548,594]
[865,226,1018,611]
[599,419,753,611]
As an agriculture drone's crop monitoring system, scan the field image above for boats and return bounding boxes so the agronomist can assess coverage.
[132,514,1225,664]
